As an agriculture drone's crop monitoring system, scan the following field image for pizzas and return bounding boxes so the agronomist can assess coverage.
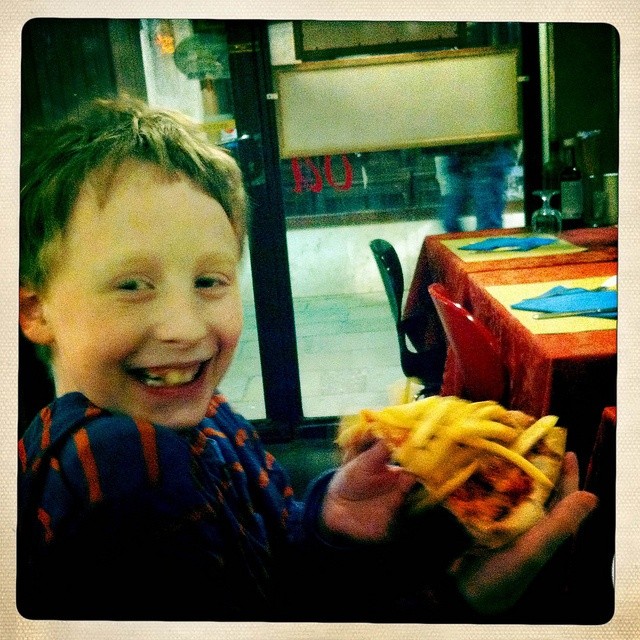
[332,394,568,550]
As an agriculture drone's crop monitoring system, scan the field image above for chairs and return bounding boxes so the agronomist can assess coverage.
[425,279,503,404]
[577,404,616,545]
[370,239,442,402]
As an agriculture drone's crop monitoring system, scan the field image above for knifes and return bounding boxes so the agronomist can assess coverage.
[532,306,617,323]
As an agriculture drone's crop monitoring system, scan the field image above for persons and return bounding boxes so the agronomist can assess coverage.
[421,140,523,234]
[20,93,599,622]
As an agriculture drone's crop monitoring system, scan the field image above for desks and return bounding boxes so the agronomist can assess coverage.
[427,229,617,364]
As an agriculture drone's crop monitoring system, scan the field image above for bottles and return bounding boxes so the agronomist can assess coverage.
[560,146,584,228]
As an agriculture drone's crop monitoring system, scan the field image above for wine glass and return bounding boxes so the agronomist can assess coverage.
[531,189,563,234]
